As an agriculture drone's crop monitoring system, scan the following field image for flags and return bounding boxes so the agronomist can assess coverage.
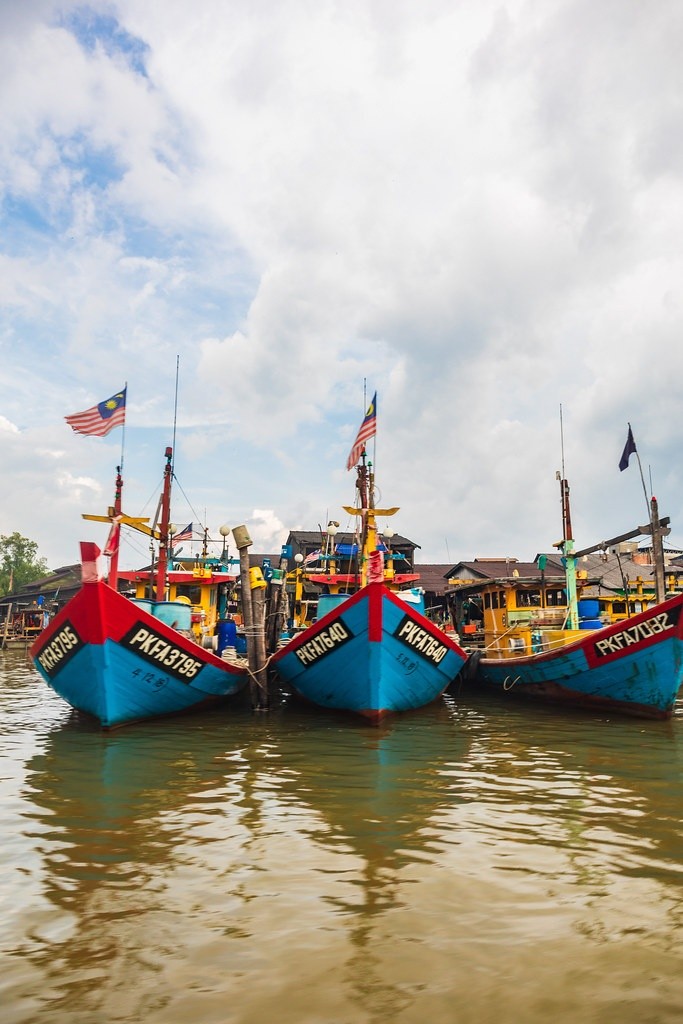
[345,389,376,472]
[62,388,126,438]
[303,548,321,564]
[619,425,637,472]
[171,522,192,548]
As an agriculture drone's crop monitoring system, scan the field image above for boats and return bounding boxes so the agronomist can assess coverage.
[270,377,471,726]
[456,402,682,721]
[28,353,252,734]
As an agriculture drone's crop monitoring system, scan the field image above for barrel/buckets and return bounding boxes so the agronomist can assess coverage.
[406,589,424,619]
[217,620,247,657]
[129,598,191,630]
[578,599,603,629]
[317,594,351,623]
